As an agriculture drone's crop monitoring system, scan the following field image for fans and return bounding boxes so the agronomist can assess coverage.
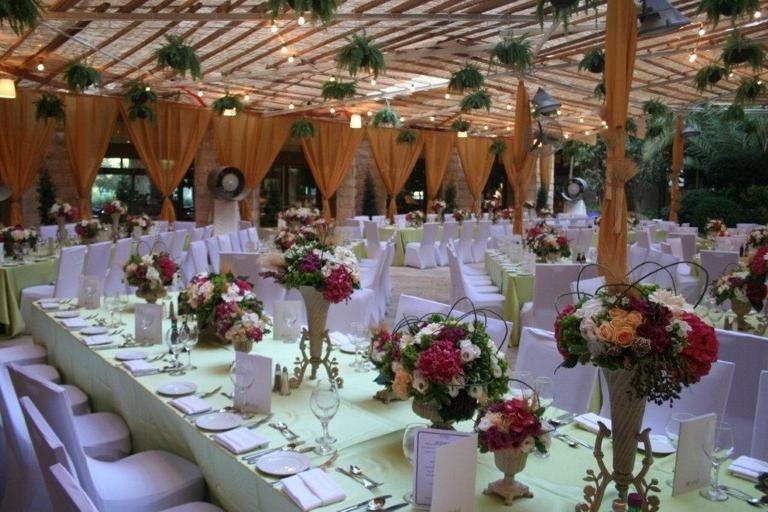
[524,117,563,156]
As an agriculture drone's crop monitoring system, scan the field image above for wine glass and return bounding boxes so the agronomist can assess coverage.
[167,323,199,377]
[229,359,256,418]
[103,288,129,329]
[401,423,428,504]
[283,306,299,345]
[534,376,555,460]
[311,381,341,455]
[138,309,156,348]
[664,412,734,502]
[349,323,371,374]
[82,278,99,309]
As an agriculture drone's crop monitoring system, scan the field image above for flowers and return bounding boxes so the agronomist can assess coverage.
[523,200,536,208]
[432,200,447,213]
[106,201,133,214]
[48,203,77,220]
[484,200,496,209]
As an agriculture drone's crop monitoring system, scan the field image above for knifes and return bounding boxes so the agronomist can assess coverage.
[340,494,409,512]
[242,437,315,467]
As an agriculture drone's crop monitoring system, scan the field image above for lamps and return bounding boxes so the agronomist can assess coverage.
[636,1,695,63]
[537,0,601,34]
[474,86,609,140]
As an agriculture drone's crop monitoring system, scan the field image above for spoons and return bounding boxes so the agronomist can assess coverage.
[350,463,385,486]
[277,421,298,440]
[556,436,578,447]
[720,488,763,505]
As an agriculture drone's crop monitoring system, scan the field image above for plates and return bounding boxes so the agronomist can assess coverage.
[81,326,107,335]
[196,412,243,430]
[157,381,197,396]
[114,350,150,359]
[54,311,79,318]
[636,434,679,455]
[257,451,310,475]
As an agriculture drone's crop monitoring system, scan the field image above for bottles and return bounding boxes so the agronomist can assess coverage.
[161,300,174,320]
[169,317,189,354]
[272,363,292,396]
[624,494,647,512]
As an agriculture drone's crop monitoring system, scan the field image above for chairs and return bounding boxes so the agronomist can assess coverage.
[0,209,767,512]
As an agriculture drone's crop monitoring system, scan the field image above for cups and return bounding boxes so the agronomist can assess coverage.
[510,371,533,397]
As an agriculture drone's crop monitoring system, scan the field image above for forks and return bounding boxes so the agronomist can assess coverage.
[335,467,376,488]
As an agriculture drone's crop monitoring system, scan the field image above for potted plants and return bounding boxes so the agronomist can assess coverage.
[447,56,481,94]
[265,0,418,145]
[485,31,537,79]
[458,89,490,117]
[577,42,606,72]
[451,116,474,131]
[694,1,768,105]
[0,0,242,129]
